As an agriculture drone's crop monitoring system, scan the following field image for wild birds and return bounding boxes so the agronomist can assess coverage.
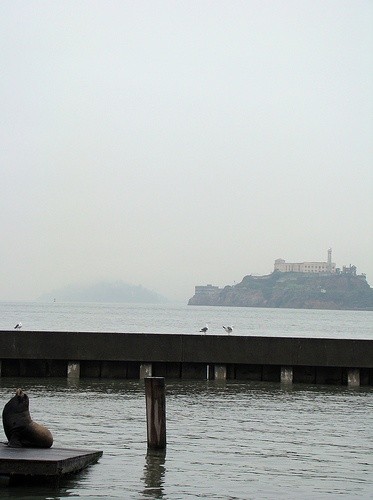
[223,324,234,335]
[13,322,24,331]
[199,325,209,335]
